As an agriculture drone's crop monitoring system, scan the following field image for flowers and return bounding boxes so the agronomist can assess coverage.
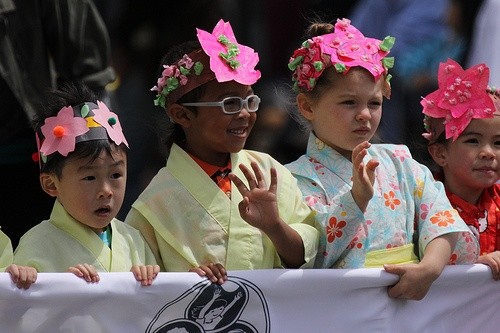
[312,18,396,84]
[420,57,497,142]
[287,39,324,92]
[149,54,204,109]
[32,100,130,170]
[196,19,262,85]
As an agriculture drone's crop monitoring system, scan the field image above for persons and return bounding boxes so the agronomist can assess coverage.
[12,83,160,285]
[0,230,37,290]
[420,58,500,280]
[123,19,321,284]
[0,0,500,255]
[282,18,480,300]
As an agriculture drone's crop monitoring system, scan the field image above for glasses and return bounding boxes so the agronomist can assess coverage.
[178,95,262,115]
